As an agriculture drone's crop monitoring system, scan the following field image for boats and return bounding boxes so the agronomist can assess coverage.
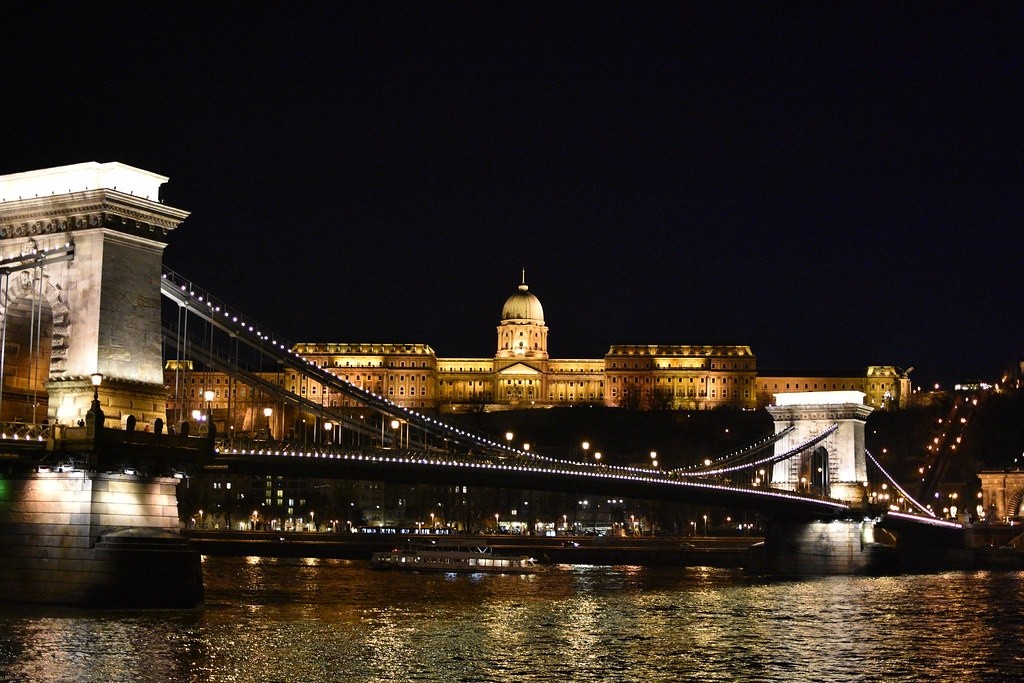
[367,549,492,571]
[389,545,547,574]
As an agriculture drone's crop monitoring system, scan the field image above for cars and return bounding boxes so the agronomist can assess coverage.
[31,459,189,481]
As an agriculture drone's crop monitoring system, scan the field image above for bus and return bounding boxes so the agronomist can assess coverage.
[499,521,529,534]
[356,524,459,534]
[573,520,616,535]
[542,522,568,531]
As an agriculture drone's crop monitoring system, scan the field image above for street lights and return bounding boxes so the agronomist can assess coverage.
[309,511,315,521]
[563,515,567,522]
[390,419,400,451]
[506,432,514,448]
[631,515,634,532]
[494,513,499,528]
[201,391,214,434]
[85,372,106,427]
[594,452,602,464]
[263,408,273,435]
[690,521,697,536]
[330,519,339,532]
[347,521,352,531]
[582,442,589,463]
[702,514,710,538]
[429,512,435,530]
[323,421,333,453]
